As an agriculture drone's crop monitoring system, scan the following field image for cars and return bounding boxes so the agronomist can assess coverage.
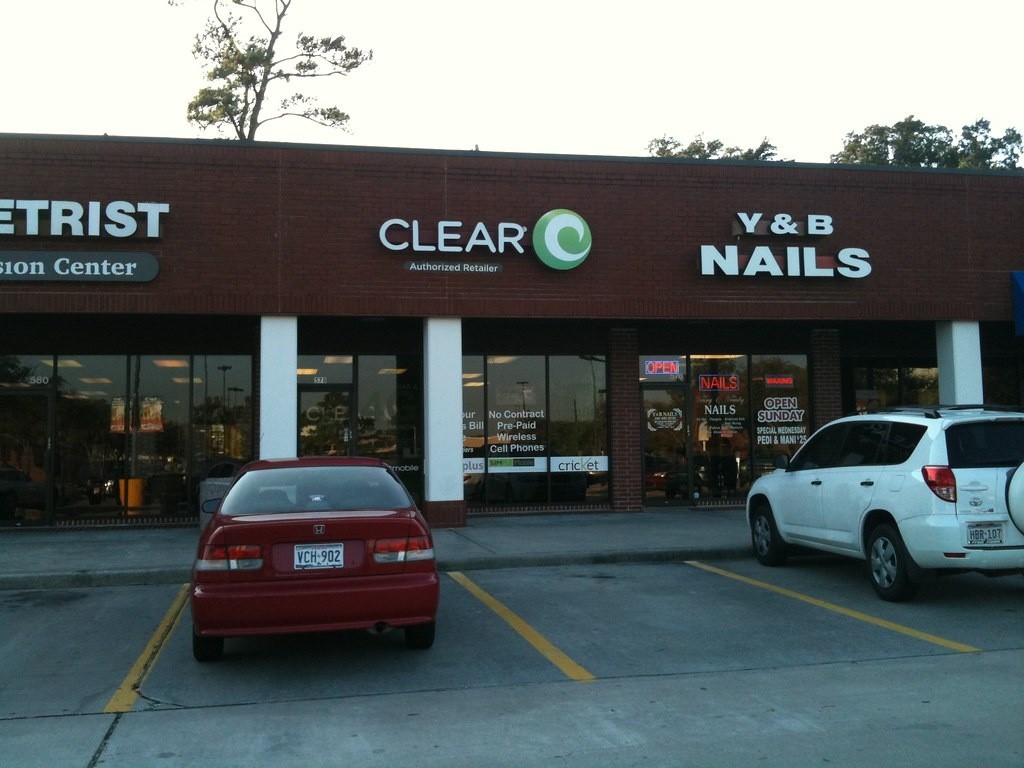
[189,456,440,661]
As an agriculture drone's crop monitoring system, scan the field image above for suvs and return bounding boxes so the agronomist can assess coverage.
[746,404,1024,603]
